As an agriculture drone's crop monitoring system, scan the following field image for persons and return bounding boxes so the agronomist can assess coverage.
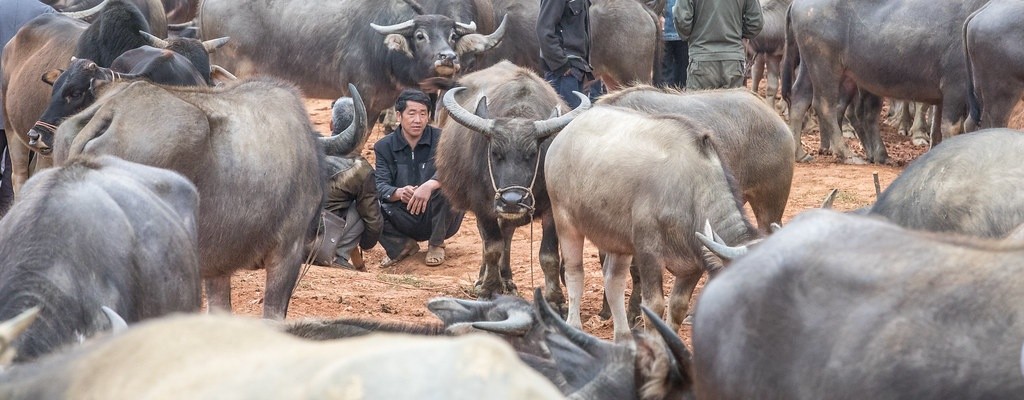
[371,89,466,272]
[535,0,596,110]
[672,0,764,91]
[662,0,690,90]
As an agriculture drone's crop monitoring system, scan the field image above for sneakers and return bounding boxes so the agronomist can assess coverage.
[333,256,355,270]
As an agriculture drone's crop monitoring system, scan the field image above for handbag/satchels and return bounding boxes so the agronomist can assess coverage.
[582,70,607,103]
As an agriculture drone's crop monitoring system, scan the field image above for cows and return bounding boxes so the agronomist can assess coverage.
[1,0,1024,400]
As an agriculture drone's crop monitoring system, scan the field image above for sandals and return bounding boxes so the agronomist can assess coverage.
[425,243,446,265]
[379,239,420,269]
